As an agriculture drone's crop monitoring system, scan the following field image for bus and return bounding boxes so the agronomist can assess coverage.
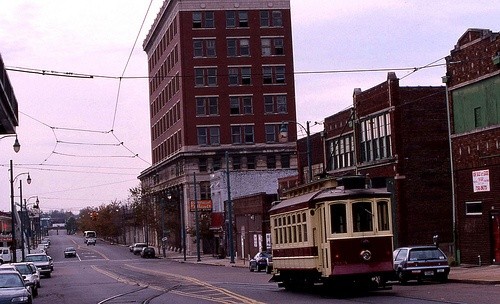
[267,175,396,293]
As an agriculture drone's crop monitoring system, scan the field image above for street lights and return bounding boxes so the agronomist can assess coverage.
[279,121,323,185]
[9,160,31,263]
[208,150,237,264]
[160,192,172,257]
[19,179,41,263]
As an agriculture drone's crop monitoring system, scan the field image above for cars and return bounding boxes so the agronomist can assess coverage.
[0,247,10,263]
[249,251,272,273]
[83,231,96,246]
[129,243,147,254]
[64,246,76,257]
[33,237,50,254]
[0,263,41,304]
[140,247,155,258]
[24,253,53,279]
[394,247,449,283]
